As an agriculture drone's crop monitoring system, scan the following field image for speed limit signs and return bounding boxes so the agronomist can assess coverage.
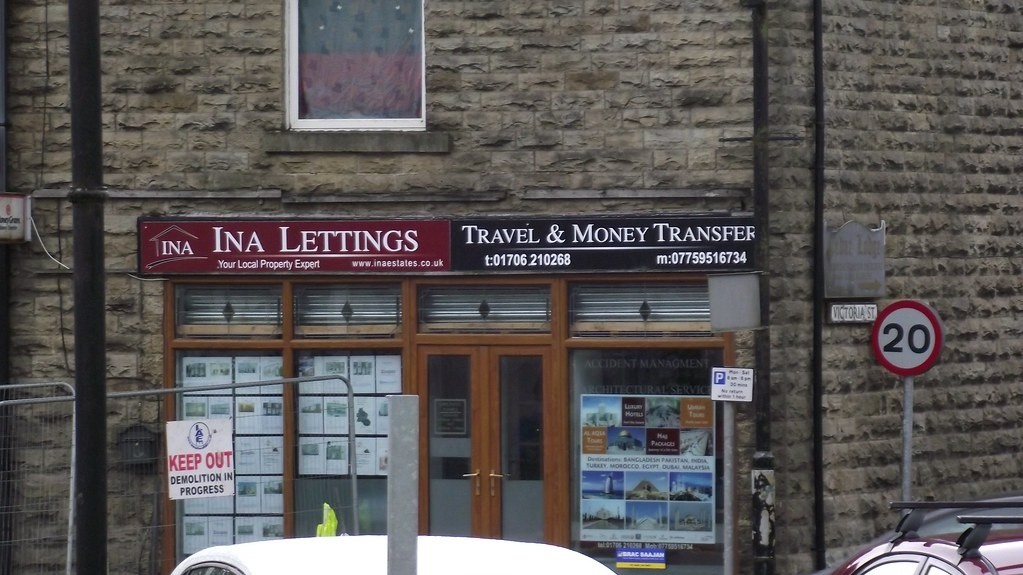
[871,301,943,377]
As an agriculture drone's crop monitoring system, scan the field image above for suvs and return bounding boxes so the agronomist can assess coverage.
[816,497,1023,575]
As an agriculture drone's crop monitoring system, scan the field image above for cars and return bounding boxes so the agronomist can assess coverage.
[169,535,615,575]
